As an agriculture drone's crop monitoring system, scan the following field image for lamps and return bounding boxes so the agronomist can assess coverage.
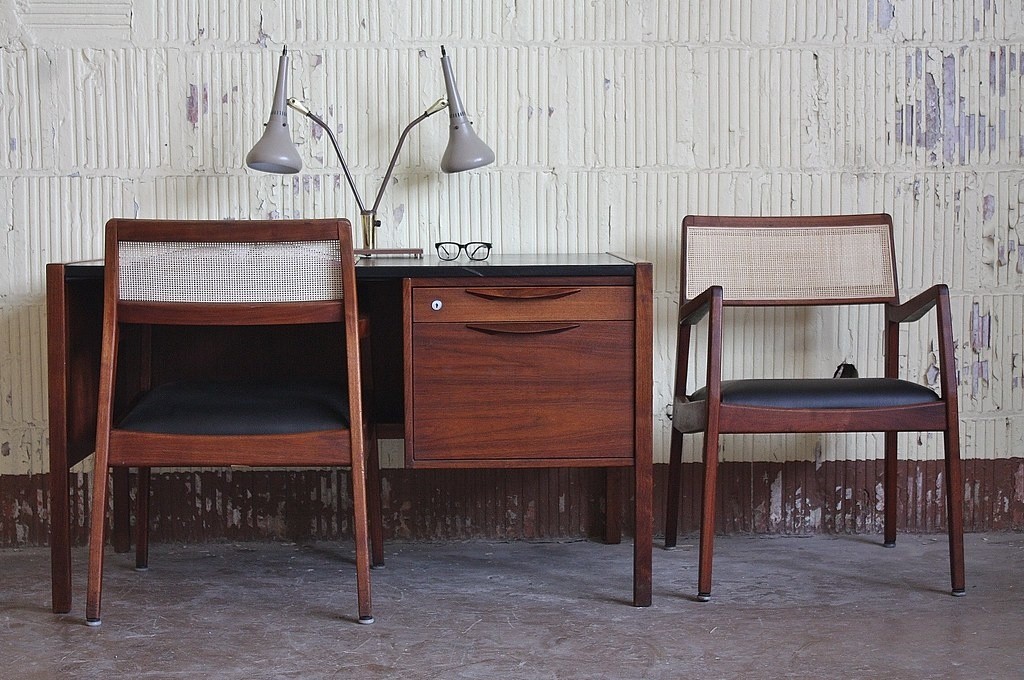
[248,43,496,262]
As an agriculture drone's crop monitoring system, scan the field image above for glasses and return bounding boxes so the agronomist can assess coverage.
[435,242,492,261]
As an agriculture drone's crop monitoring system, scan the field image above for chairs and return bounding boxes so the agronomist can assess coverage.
[84,219,383,626]
[671,214,964,601]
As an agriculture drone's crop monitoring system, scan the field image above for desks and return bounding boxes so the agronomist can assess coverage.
[45,253,655,607]
